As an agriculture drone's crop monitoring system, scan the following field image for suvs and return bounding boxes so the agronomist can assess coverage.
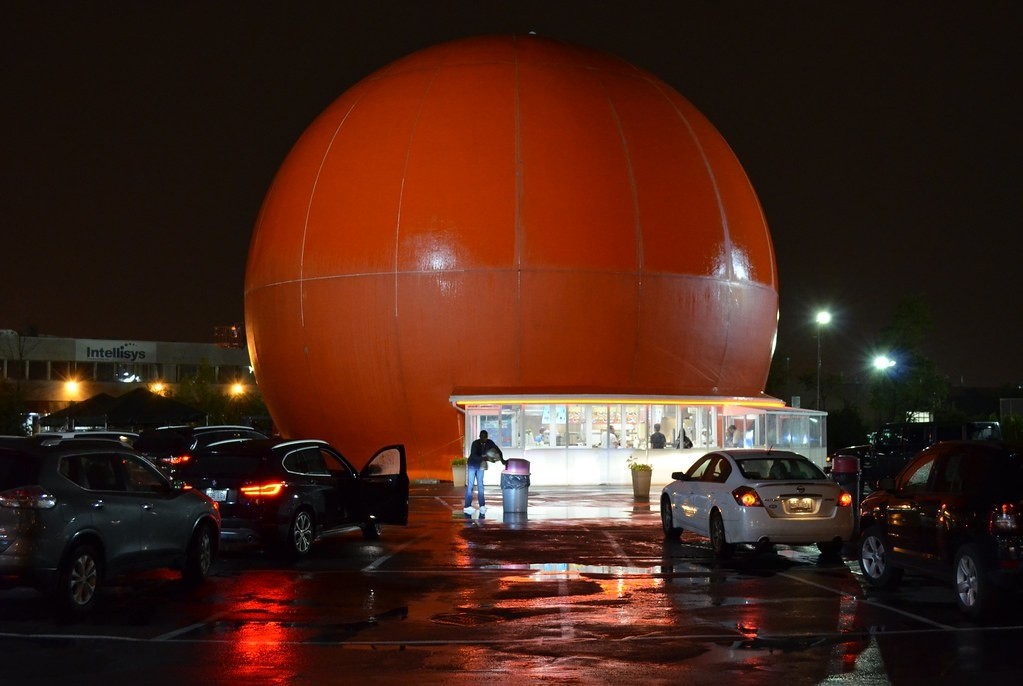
[0,417,272,612]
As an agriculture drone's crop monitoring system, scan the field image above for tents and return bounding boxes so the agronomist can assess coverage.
[39,387,134,432]
[104,387,208,429]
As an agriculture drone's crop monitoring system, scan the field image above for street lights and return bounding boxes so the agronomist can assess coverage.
[817,313,831,412]
[875,355,896,426]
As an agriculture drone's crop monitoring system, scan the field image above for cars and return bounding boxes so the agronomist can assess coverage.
[824,443,917,493]
[660,446,856,557]
[177,439,410,559]
[859,440,1023,611]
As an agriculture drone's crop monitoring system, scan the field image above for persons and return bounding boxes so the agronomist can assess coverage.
[672,428,693,449]
[650,424,666,448]
[463,430,507,510]
[536,427,545,442]
[725,425,743,447]
[360,466,380,548]
[601,426,619,448]
[701,429,713,444]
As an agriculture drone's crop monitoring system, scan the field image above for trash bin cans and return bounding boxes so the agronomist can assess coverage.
[501,458,532,513]
[830,454,861,533]
[501,512,530,570]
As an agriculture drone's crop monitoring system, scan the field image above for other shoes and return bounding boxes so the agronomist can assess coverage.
[464,506,476,511]
[480,506,486,511]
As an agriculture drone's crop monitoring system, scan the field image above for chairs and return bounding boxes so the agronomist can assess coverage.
[746,472,761,480]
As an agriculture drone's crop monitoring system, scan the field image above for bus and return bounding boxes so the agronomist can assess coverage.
[874,420,1000,447]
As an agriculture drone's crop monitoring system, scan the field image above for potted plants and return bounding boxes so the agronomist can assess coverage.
[452,457,468,486]
[629,464,652,498]
